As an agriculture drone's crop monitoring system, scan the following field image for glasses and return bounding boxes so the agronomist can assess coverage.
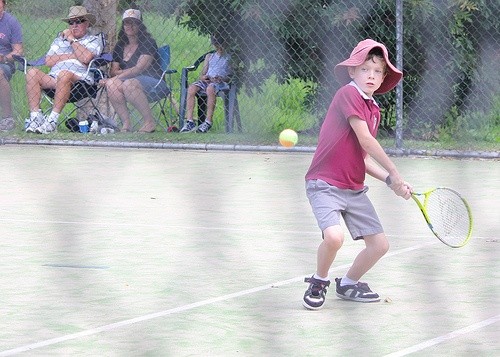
[67,19,88,25]
[123,20,138,24]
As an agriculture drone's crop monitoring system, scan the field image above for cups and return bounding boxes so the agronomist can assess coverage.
[79,123,89,133]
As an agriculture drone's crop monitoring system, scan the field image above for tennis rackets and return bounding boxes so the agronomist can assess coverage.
[385,176,473,248]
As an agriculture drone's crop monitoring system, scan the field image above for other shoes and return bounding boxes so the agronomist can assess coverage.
[137,130,155,133]
[119,129,134,133]
[0,117,16,132]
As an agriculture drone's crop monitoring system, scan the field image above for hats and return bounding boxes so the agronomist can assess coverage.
[334,39,403,95]
[61,5,96,27]
[121,9,142,23]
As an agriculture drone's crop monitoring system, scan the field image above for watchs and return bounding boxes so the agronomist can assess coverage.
[71,39,79,45]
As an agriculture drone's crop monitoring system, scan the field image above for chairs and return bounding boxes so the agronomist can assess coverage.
[113,46,177,131]
[177,49,249,134]
[12,32,111,128]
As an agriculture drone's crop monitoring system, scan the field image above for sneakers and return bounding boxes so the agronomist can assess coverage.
[195,121,213,134]
[25,116,46,133]
[35,116,58,135]
[302,273,331,311]
[334,277,381,303]
[178,120,197,134]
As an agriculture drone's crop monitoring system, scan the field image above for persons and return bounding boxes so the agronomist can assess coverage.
[180,31,237,133]
[303,39,416,311]
[0,0,27,131]
[98,10,169,131]
[26,6,104,135]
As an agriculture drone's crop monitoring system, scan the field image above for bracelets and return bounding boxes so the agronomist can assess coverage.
[386,174,392,186]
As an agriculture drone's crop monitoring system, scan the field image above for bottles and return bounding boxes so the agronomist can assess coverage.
[89,119,98,135]
[101,127,116,135]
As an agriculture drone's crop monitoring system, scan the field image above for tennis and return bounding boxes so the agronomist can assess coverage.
[279,129,298,147]
